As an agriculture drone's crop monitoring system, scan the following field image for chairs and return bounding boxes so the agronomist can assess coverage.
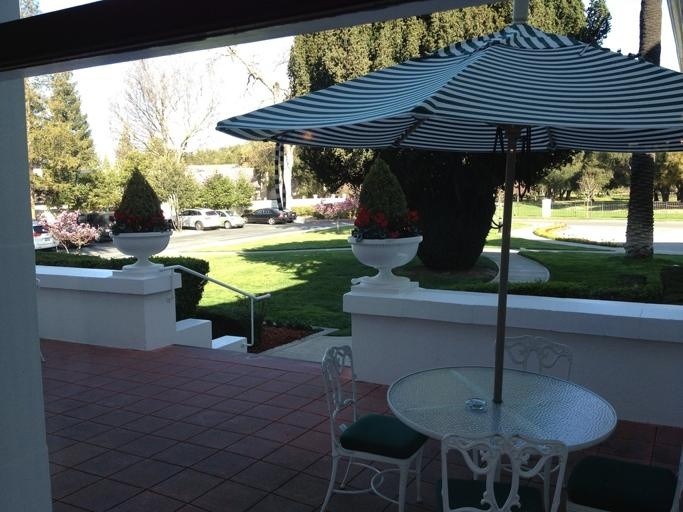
[439,434,565,511]
[478,335,572,486]
[567,449,683,510]
[322,344,428,511]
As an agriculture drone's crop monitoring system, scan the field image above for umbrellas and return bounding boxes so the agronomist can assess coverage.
[212,23,683,404]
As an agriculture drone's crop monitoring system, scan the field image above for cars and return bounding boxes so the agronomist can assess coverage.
[32,211,116,251]
[215,209,245,230]
[241,207,296,225]
[179,207,223,231]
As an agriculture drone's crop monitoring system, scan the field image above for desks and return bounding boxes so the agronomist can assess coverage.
[387,363,616,479]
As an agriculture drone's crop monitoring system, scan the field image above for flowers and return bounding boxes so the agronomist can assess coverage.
[106,167,175,237]
[351,152,421,243]
